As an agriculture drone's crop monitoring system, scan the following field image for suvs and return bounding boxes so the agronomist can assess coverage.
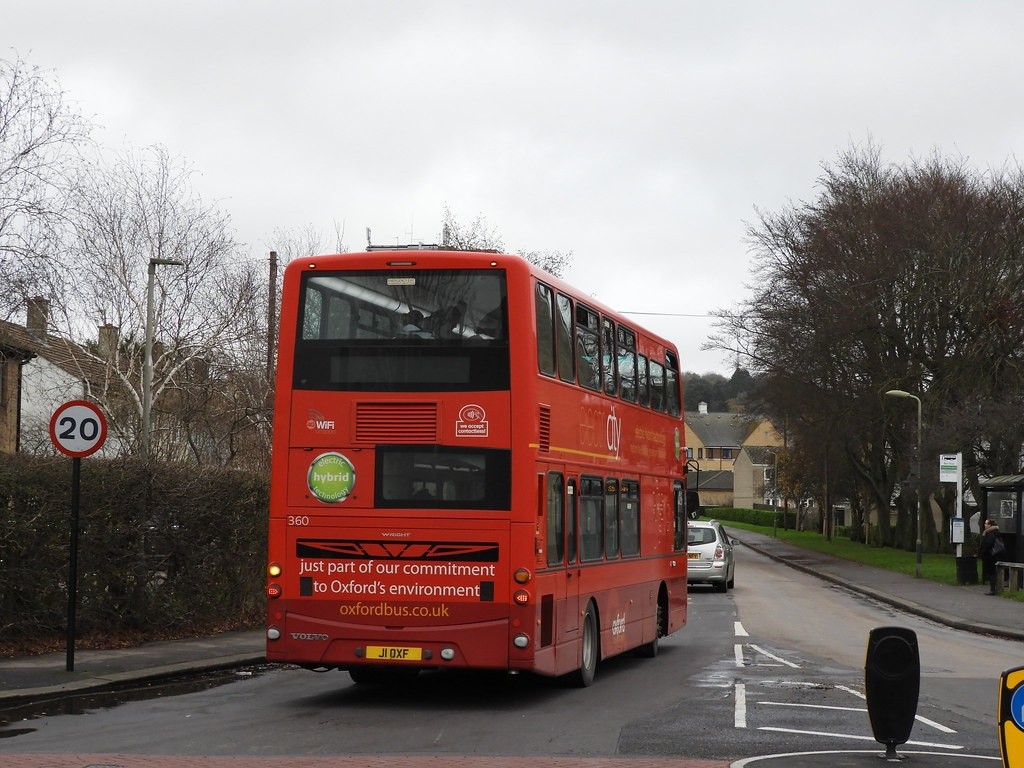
[686,520,735,592]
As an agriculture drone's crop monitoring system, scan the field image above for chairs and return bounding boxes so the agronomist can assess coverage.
[687,535,695,541]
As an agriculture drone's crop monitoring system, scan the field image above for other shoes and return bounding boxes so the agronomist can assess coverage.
[984,591,996,595]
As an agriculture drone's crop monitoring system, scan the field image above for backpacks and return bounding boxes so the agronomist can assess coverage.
[991,532,1006,559]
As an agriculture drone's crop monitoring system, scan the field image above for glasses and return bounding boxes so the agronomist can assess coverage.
[984,523,987,524]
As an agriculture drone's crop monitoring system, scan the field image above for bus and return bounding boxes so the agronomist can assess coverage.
[264,242,700,690]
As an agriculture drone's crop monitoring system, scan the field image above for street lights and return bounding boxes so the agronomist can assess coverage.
[764,449,778,535]
[885,390,923,575]
[133,255,186,627]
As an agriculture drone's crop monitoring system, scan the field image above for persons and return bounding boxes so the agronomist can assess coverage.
[393,309,435,339]
[431,305,467,339]
[978,519,1006,595]
